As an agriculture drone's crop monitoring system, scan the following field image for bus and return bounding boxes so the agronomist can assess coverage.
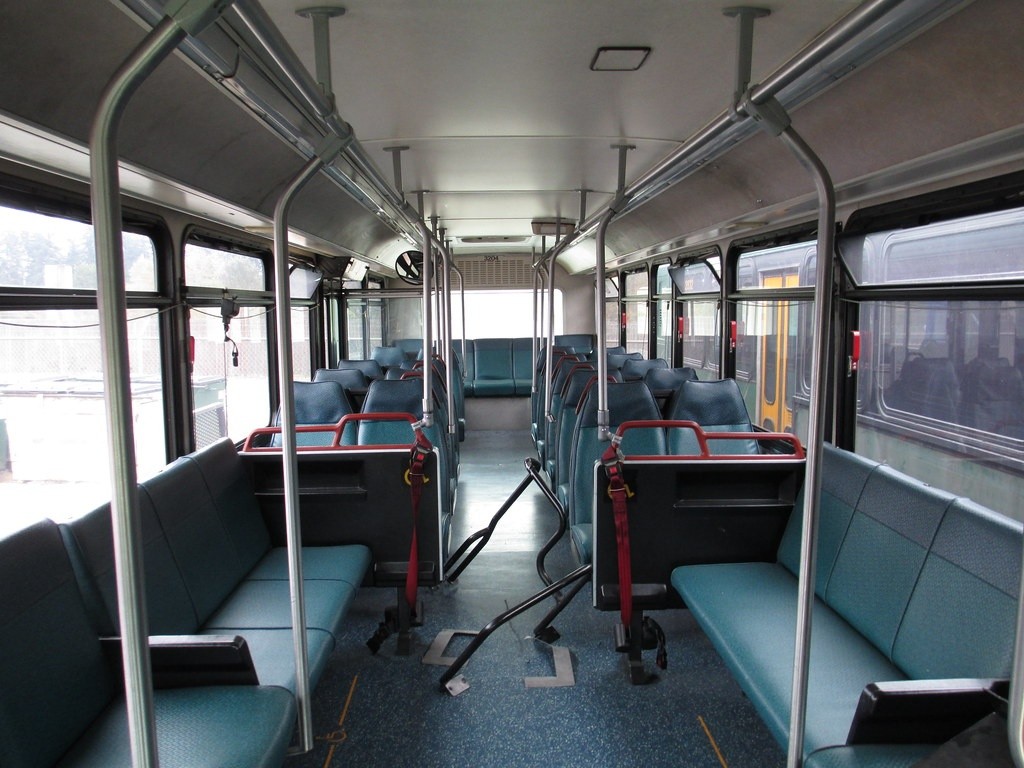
[606,207,1024,523]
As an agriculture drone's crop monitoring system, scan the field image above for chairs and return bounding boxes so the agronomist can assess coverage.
[267,346,761,569]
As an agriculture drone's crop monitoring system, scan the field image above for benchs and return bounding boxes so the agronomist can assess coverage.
[389,334,598,396]
[0,435,370,768]
[676,440,1024,768]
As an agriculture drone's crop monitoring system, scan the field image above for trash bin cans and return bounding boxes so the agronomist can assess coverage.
[0,369,227,481]
